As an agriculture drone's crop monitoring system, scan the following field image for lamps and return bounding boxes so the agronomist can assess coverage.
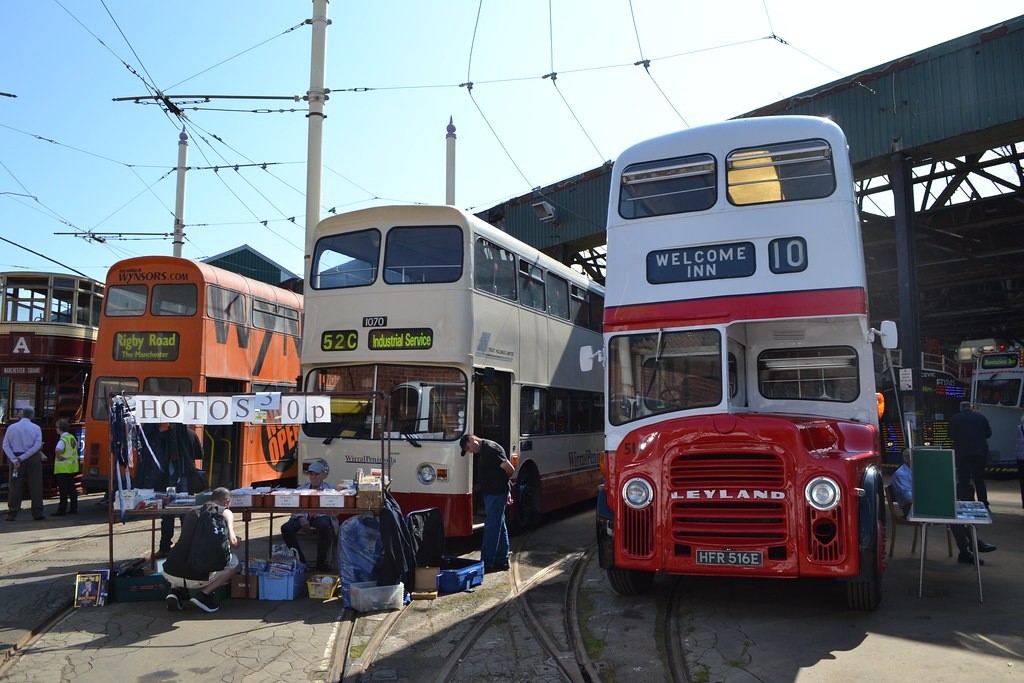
[532,201,557,222]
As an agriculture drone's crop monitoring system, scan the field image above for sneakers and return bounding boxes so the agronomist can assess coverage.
[190,592,219,612]
[165,590,183,610]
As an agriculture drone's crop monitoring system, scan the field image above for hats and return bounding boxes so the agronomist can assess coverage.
[306,462,325,474]
[460,434,472,456]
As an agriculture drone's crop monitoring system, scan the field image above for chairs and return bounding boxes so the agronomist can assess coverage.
[884,485,954,558]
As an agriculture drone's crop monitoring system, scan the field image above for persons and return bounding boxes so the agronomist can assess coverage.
[281,459,343,571]
[947,401,992,513]
[50,419,80,516]
[2,406,45,521]
[151,423,204,559]
[1015,414,1024,509]
[161,486,240,613]
[79,579,96,605]
[892,448,997,565]
[460,435,516,573]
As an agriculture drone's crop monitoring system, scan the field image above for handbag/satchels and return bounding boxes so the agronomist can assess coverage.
[187,468,207,492]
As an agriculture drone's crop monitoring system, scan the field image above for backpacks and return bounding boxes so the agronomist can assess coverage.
[187,502,231,571]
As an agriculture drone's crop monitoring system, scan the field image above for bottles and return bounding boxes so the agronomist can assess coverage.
[12,468,18,480]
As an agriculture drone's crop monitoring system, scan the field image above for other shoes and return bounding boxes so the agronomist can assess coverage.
[958,553,984,565]
[4,517,15,521]
[485,564,497,571]
[34,515,45,520]
[316,564,330,571]
[969,541,996,552]
[155,550,171,558]
[50,511,66,515]
[67,511,77,515]
[497,561,509,569]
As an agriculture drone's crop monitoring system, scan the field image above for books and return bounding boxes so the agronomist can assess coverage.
[241,555,298,575]
[117,558,159,578]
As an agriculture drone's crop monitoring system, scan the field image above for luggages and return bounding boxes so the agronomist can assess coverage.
[405,507,485,592]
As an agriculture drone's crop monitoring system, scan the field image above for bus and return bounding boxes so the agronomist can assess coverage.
[0,270,106,503]
[577,116,898,611]
[80,254,302,493]
[297,204,604,551]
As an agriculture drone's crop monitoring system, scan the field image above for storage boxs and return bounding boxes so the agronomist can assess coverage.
[229,567,302,600]
[307,574,340,598]
[349,582,404,613]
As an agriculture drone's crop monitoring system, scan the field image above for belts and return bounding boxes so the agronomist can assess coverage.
[14,452,25,455]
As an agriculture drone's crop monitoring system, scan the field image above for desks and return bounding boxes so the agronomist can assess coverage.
[909,501,992,603]
[113,506,368,597]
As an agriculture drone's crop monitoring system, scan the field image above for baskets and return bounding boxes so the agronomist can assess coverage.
[306,575,338,598]
